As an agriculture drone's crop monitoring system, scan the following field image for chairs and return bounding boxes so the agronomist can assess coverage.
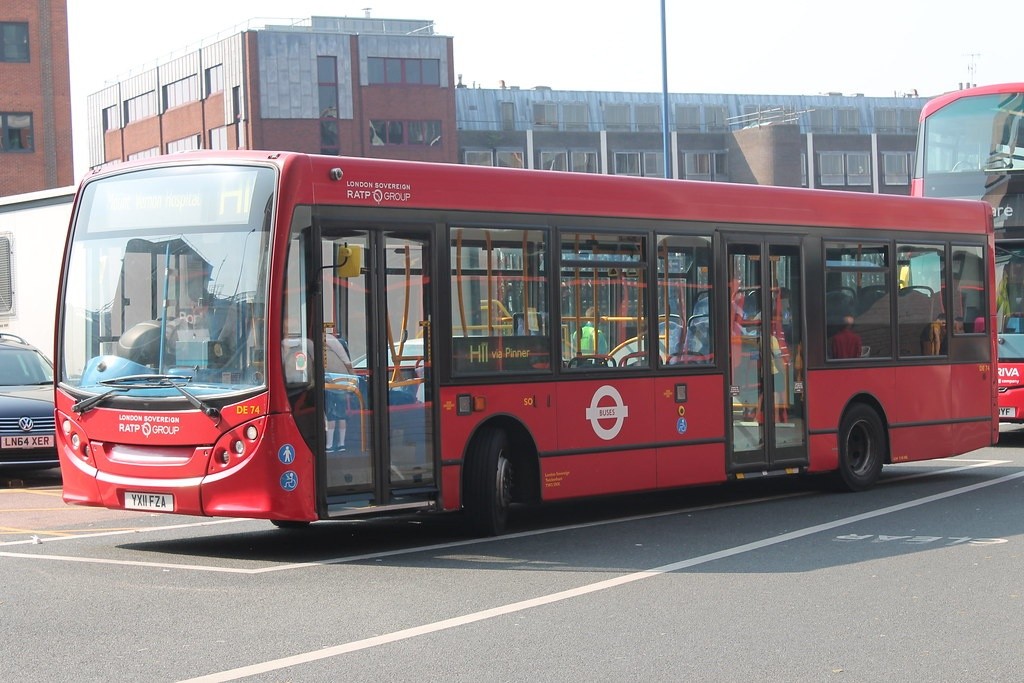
[509,312,715,369]
[829,284,935,323]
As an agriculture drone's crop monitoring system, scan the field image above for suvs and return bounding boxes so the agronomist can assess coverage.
[0,333,60,471]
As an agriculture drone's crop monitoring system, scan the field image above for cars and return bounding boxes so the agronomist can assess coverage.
[352,338,424,401]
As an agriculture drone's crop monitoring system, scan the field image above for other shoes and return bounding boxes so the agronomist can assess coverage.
[326,445,334,453]
[333,443,346,451]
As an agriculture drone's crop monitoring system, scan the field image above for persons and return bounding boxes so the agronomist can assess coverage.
[572,307,608,364]
[831,315,862,358]
[919,312,964,356]
[734,283,790,424]
[322,316,359,453]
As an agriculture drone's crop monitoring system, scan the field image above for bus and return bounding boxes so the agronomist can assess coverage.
[911,82,1024,424]
[53,149,999,531]
